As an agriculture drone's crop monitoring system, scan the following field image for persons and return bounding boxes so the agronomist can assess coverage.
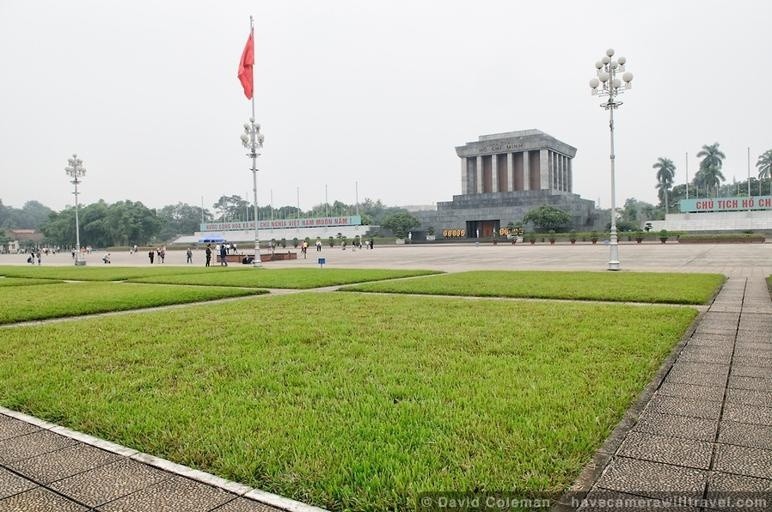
[205,244,211,266]
[36,249,41,265]
[71,248,76,259]
[186,248,193,263]
[133,243,139,255]
[216,242,238,266]
[303,241,308,253]
[86,246,90,255]
[407,231,412,240]
[89,246,93,255]
[352,238,374,250]
[149,249,155,264]
[103,253,111,263]
[81,246,86,256]
[161,249,165,263]
[157,247,161,263]
[341,239,346,250]
[27,253,36,264]
[315,239,322,252]
[271,239,276,255]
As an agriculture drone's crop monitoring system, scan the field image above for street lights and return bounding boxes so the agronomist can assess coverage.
[589,49,633,271]
[240,116,264,270]
[65,153,86,265]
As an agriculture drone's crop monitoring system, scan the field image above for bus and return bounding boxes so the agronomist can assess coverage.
[198,238,225,243]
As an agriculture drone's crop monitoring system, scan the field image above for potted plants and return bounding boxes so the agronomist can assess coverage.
[271,237,360,249]
[568,229,578,243]
[659,230,668,243]
[426,226,435,240]
[547,230,558,244]
[635,229,645,243]
[528,230,537,244]
[590,231,600,244]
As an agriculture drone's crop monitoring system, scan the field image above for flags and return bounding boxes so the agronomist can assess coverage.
[236,28,255,101]
